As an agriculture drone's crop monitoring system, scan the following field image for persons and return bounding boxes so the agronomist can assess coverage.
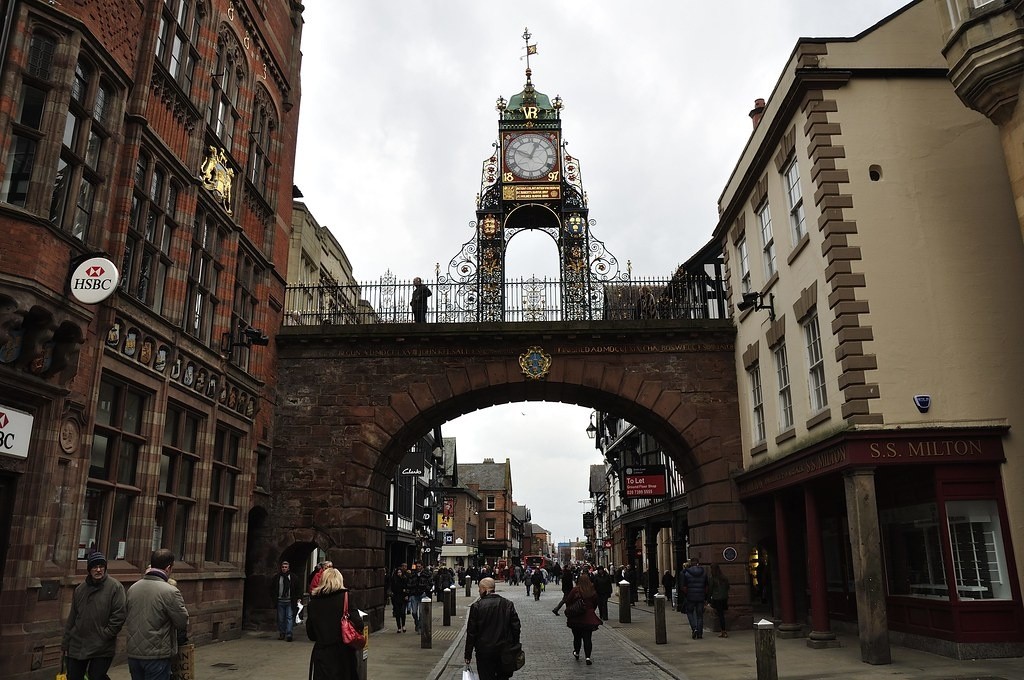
[410,277,432,323]
[464,577,522,680]
[271,561,303,642]
[683,557,709,639]
[126,547,190,680]
[383,560,456,634]
[707,562,731,638]
[306,560,333,601]
[522,564,553,601]
[635,285,657,320]
[60,551,127,680]
[662,570,674,601]
[565,575,604,665]
[305,568,365,680]
[457,563,524,588]
[552,562,659,621]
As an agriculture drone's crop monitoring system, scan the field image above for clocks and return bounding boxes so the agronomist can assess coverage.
[506,133,557,180]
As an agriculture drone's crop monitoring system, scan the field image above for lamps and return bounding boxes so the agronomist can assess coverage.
[222,328,270,359]
[586,410,596,438]
[737,291,776,321]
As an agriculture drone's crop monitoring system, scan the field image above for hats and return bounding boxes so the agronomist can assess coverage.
[87,550,107,571]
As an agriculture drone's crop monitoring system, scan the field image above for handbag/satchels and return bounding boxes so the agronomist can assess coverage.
[504,643,525,671]
[463,663,479,680]
[341,591,366,649]
[564,596,586,618]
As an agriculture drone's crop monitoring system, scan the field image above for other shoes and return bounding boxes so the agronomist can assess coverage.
[718,631,728,638]
[397,628,407,633]
[552,609,561,616]
[278,632,293,642]
[586,658,593,665]
[692,630,703,640]
[573,650,580,660]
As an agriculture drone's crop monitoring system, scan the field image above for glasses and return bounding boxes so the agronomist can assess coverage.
[282,566,288,569]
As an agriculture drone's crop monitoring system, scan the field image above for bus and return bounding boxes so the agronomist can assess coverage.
[524,555,552,573]
